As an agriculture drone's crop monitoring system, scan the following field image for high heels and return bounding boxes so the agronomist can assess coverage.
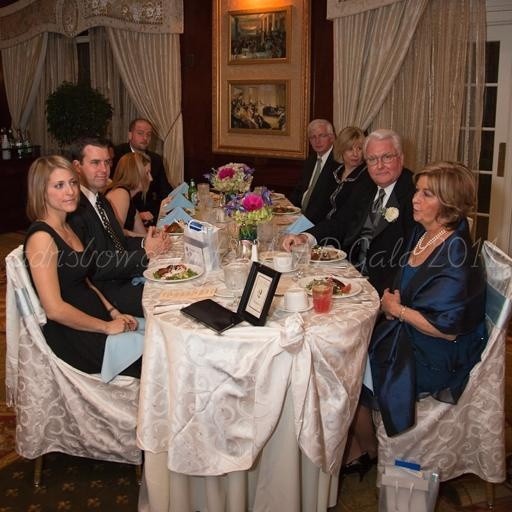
[340,450,372,483]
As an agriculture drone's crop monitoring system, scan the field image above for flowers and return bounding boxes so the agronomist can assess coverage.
[229,192,272,240]
[383,207,400,223]
[208,163,254,208]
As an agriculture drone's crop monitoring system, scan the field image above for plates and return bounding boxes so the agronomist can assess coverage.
[213,286,241,298]
[142,263,205,284]
[310,247,348,264]
[271,297,314,313]
[278,268,299,273]
[297,275,363,298]
[168,232,184,235]
[273,206,301,215]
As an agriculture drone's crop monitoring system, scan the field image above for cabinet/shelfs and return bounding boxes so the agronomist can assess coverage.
[0,145,41,234]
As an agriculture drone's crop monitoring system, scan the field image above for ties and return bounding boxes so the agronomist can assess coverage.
[95,195,127,265]
[301,157,323,214]
[371,188,386,227]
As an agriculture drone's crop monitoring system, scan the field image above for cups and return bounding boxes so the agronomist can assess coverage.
[257,220,276,262]
[284,287,309,310]
[274,253,293,271]
[313,274,334,314]
[198,183,210,210]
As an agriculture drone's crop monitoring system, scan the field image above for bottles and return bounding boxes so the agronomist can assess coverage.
[0,128,4,143]
[23,128,33,155]
[2,135,11,160]
[188,177,197,206]
[14,128,24,159]
[8,129,15,148]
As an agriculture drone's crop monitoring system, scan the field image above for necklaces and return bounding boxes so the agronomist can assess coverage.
[413,226,448,256]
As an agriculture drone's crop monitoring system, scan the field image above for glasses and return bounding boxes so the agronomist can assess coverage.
[365,151,398,167]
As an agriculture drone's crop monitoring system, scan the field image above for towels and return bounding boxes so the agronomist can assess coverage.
[101,316,145,383]
[132,276,145,287]
[286,215,316,236]
[161,183,194,230]
[363,355,375,396]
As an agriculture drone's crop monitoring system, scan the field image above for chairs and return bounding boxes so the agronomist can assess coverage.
[369,241,512,508]
[5,245,142,488]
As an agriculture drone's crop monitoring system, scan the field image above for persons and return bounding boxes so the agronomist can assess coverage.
[21,153,145,474]
[303,124,367,233]
[108,116,175,229]
[97,138,116,196]
[105,149,149,237]
[276,127,420,299]
[291,118,341,224]
[340,159,490,484]
[64,136,174,318]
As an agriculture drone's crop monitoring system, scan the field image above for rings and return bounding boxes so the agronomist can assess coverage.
[380,298,383,303]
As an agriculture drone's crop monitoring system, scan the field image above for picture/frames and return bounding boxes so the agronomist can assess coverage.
[211,0,311,161]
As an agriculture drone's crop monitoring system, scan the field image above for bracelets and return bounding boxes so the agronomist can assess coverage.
[108,306,116,314]
[399,305,408,323]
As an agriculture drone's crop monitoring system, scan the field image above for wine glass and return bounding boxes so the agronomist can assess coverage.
[224,263,248,307]
[291,243,310,282]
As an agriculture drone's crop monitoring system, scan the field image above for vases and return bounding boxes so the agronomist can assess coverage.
[239,221,257,240]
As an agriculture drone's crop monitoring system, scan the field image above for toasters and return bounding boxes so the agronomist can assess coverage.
[251,239,259,262]
